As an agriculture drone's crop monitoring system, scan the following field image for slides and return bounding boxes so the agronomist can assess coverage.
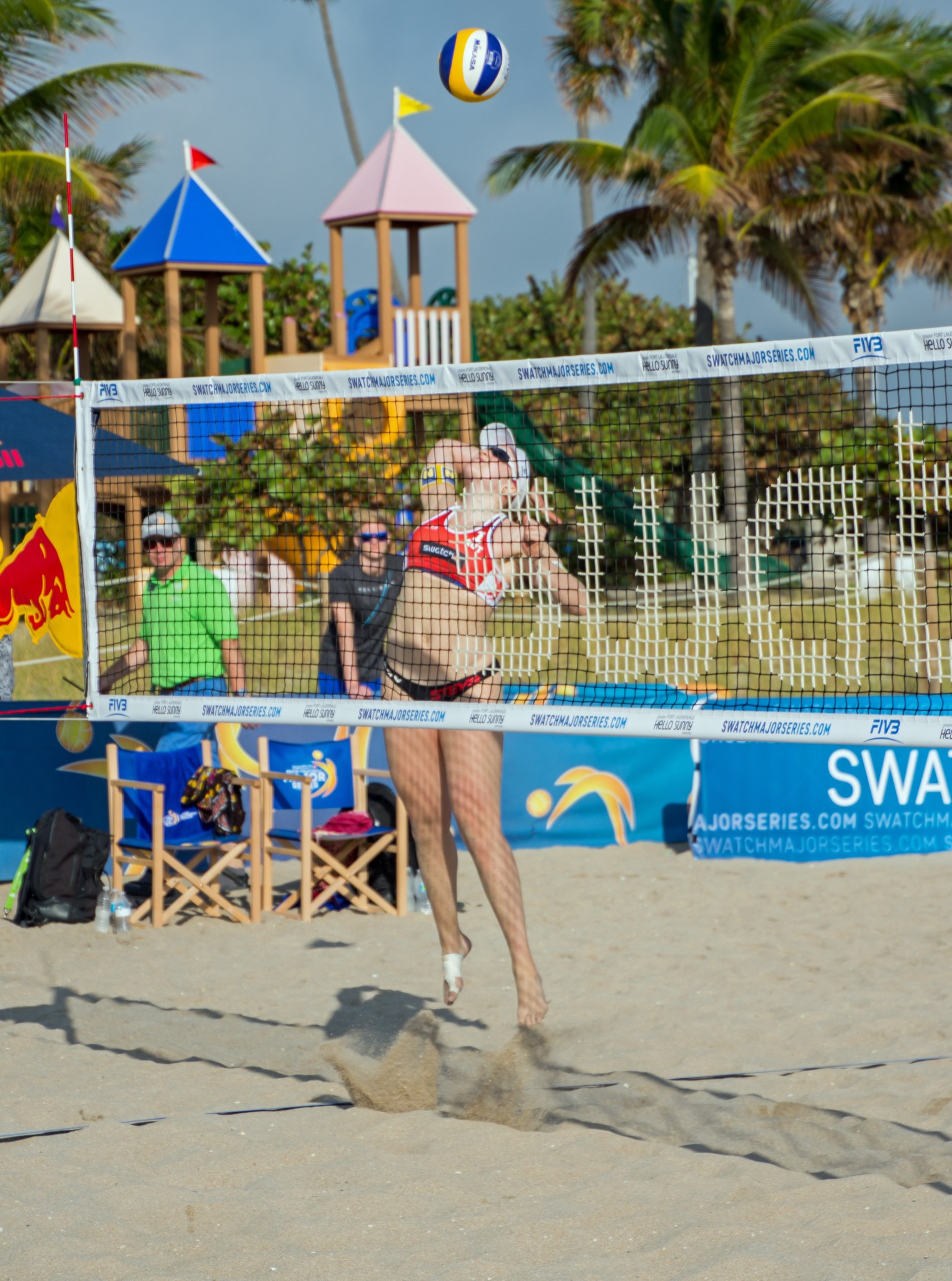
[468,328,803,591]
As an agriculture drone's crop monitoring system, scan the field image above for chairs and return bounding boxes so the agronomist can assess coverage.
[97,739,268,930]
[255,732,413,933]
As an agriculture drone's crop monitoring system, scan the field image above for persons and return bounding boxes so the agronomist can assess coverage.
[84,514,257,900]
[376,422,589,1029]
[316,513,410,695]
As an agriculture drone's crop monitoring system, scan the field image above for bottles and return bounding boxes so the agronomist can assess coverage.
[115,892,134,944]
[110,888,120,934]
[415,869,433,915]
[408,866,415,912]
[93,887,111,933]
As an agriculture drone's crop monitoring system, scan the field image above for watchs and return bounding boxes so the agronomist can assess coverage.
[233,688,249,697]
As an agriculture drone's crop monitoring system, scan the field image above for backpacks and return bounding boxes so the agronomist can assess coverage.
[13,807,110,928]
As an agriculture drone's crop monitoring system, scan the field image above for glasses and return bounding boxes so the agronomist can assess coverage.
[358,532,388,541]
[143,535,180,549]
[486,444,517,484]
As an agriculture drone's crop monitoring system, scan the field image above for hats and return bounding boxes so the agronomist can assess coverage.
[140,511,180,539]
[479,422,530,512]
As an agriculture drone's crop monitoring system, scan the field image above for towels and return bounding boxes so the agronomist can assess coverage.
[179,763,247,838]
[130,740,214,841]
[297,808,375,845]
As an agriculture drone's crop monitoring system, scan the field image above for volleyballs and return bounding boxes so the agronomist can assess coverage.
[439,28,509,102]
